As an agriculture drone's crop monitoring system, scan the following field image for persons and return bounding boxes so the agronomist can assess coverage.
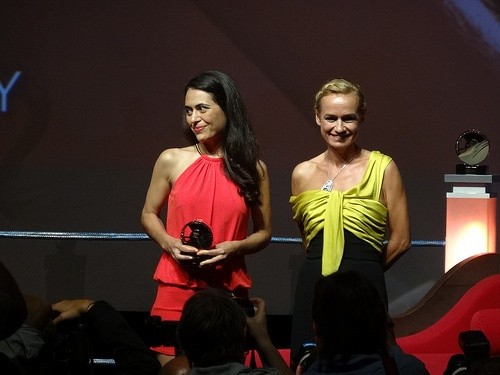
[1,265,162,374]
[173,285,292,375]
[294,265,431,374]
[139,69,274,347]
[289,78,412,352]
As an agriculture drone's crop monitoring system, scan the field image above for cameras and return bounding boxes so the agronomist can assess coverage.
[41,310,90,366]
[294,343,318,374]
[234,297,255,318]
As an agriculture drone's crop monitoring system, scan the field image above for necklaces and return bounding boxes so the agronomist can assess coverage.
[320,150,359,192]
